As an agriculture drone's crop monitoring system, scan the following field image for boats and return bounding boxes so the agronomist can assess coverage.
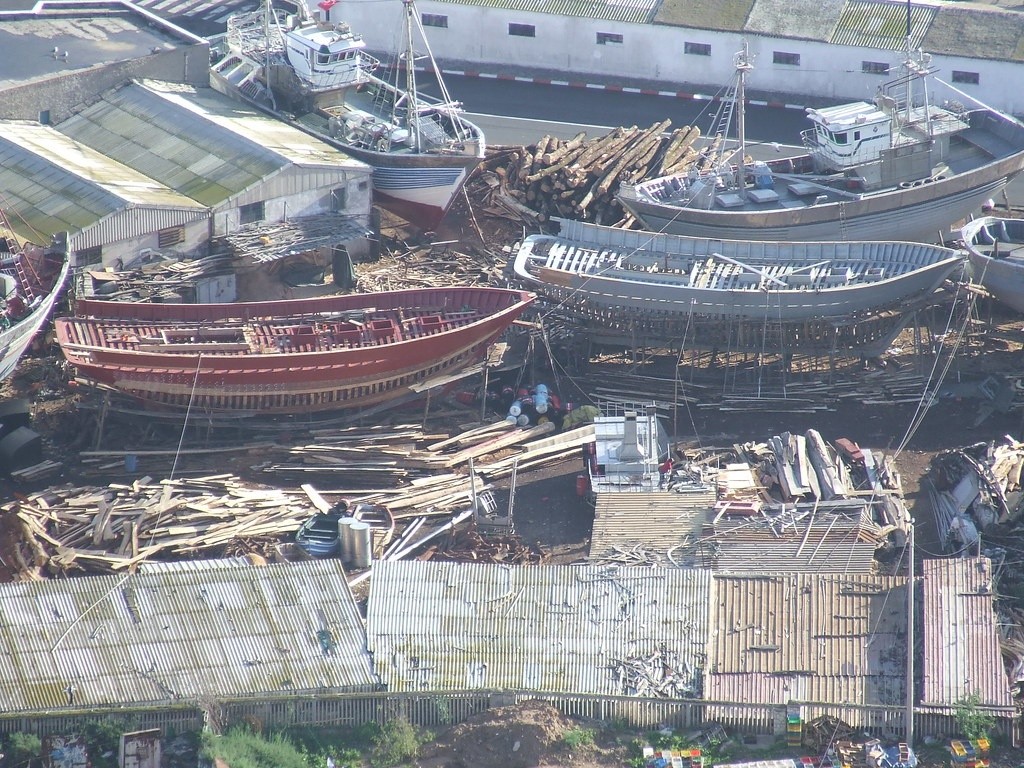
[958,214,1024,312]
[293,499,395,564]
[53,283,541,424]
[609,0,1024,260]
[514,214,966,365]
[208,0,491,235]
[0,196,76,392]
